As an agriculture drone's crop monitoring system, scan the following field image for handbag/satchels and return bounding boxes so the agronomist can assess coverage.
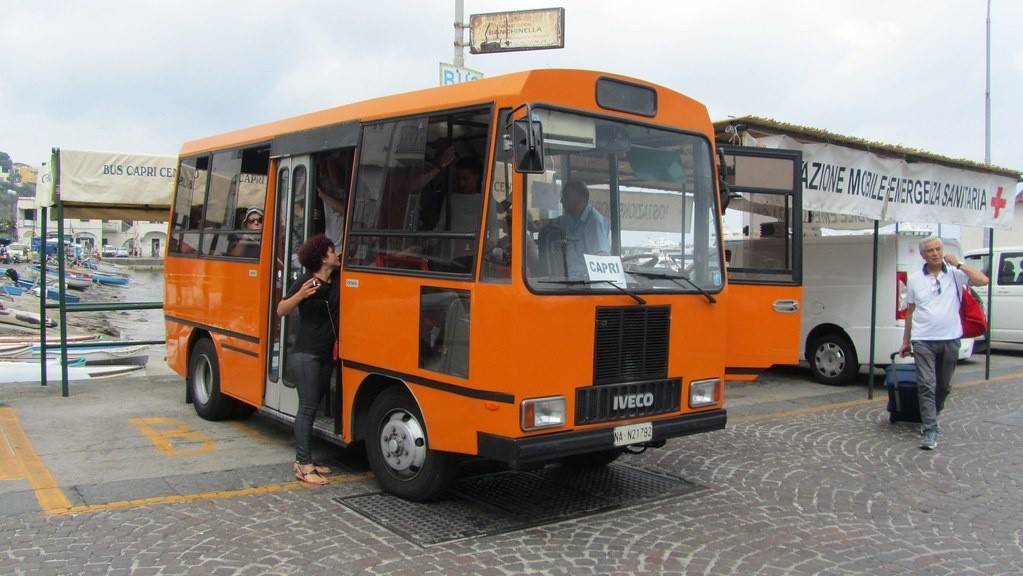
[952,269,988,338]
[324,300,338,361]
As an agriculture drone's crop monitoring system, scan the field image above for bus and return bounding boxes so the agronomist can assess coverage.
[162,67,744,500]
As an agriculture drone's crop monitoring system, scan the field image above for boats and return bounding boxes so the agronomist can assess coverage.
[0,253,149,382]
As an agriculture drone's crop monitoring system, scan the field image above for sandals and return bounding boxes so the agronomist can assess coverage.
[295,463,330,485]
[292,460,300,472]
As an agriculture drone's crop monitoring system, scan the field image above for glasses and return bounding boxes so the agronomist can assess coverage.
[246,216,263,223]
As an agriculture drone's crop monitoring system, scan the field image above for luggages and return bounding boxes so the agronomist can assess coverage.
[884,351,922,423]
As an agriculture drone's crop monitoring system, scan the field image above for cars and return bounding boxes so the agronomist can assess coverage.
[6,243,34,262]
[961,247,1023,354]
[102,245,117,257]
[117,248,128,257]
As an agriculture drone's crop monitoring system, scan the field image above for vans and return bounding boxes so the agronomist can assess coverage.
[676,232,975,386]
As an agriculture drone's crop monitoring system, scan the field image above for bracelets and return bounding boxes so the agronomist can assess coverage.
[957,263,962,269]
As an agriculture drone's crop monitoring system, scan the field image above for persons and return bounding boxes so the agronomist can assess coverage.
[180,139,611,276]
[128,246,142,257]
[276,234,422,485]
[899,234,989,449]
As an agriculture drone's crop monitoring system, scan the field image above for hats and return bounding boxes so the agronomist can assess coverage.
[241,206,264,229]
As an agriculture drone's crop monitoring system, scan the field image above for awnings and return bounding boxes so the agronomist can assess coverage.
[526,115,1023,400]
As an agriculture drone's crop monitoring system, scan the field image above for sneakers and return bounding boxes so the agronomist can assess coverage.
[919,431,938,449]
